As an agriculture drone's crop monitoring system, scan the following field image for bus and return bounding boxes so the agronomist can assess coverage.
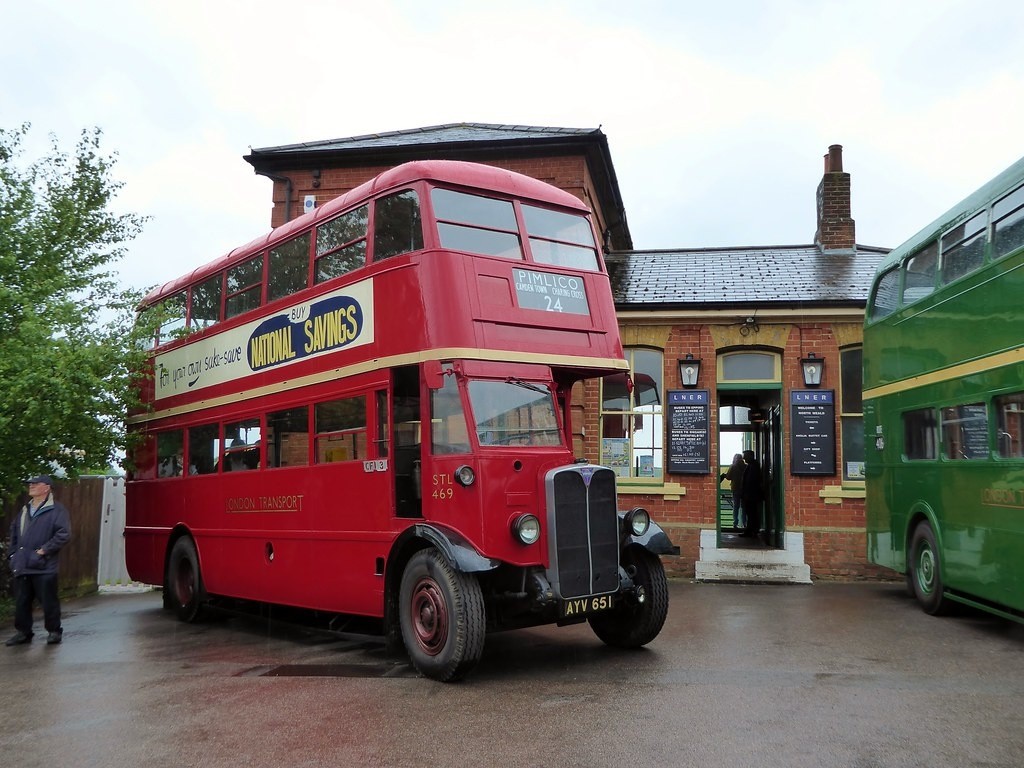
[862,157,1024,626]
[123,160,683,684]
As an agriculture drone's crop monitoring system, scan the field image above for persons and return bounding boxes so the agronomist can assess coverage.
[245,439,260,469]
[726,454,748,529]
[738,450,761,538]
[5,476,70,646]
[214,438,249,473]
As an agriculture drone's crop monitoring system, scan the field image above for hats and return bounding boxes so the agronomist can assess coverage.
[25,475,54,489]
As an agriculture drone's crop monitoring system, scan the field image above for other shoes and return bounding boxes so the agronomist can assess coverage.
[6,631,34,646]
[738,533,750,537]
[743,525,747,528]
[733,524,738,528]
[47,626,63,643]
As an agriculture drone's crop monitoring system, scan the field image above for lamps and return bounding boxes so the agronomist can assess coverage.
[677,353,701,388]
[800,351,824,386]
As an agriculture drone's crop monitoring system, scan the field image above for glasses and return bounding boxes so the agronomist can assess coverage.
[178,452,183,456]
